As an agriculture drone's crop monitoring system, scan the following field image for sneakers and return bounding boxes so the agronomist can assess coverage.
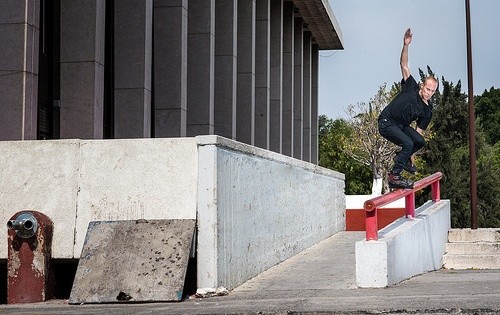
[404,161,419,176]
[388,175,414,189]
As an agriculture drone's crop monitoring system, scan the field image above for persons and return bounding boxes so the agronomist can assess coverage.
[378,27,437,188]
